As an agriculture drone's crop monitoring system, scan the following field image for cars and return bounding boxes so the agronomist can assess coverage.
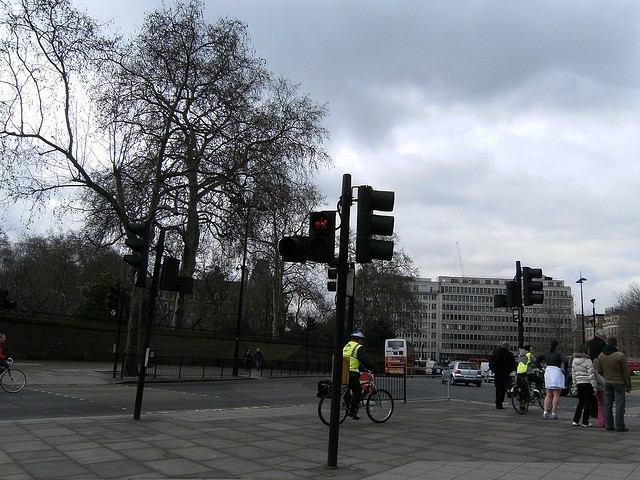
[414,358,437,375]
[442,360,482,387]
[484,368,495,383]
[627,357,639,375]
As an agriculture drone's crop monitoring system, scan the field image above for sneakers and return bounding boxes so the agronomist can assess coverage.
[543,414,550,420]
[551,414,560,420]
[582,423,593,427]
[572,422,580,426]
[616,429,628,432]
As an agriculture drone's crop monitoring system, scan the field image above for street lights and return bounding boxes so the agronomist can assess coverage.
[575,271,587,344]
[228,187,270,376]
[591,298,596,337]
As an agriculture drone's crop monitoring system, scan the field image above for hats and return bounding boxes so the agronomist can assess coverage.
[351,331,366,339]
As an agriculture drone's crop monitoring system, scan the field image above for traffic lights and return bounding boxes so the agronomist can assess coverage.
[355,185,395,263]
[279,233,307,265]
[325,259,339,292]
[122,220,149,288]
[306,208,335,264]
[523,267,544,306]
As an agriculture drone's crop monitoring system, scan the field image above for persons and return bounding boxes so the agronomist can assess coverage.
[586,330,608,361]
[244,348,254,369]
[517,344,543,411]
[598,337,631,432]
[592,359,605,426]
[346,332,370,420]
[253,348,263,369]
[572,344,598,426]
[488,341,516,409]
[535,340,568,419]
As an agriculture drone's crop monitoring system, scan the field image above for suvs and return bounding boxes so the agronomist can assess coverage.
[561,356,578,398]
[431,361,448,378]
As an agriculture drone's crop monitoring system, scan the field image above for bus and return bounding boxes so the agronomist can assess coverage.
[384,338,415,375]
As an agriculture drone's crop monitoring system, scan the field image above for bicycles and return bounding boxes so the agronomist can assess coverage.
[510,369,553,415]
[317,367,395,426]
[0,357,27,394]
